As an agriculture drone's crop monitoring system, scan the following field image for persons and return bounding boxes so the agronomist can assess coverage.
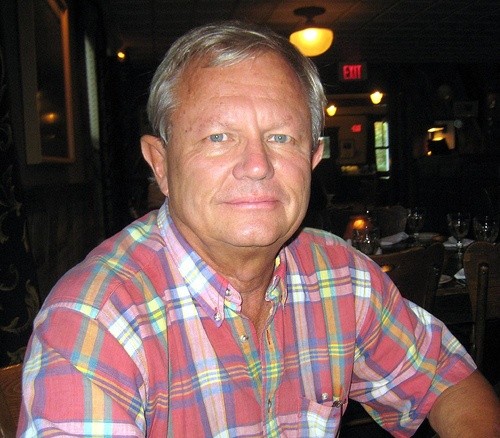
[16,20,500,438]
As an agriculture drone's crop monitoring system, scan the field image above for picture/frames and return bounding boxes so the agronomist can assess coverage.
[18,0,77,165]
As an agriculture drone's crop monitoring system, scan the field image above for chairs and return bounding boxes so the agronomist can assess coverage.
[331,207,500,373]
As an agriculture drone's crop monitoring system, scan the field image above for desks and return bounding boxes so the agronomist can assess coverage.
[378,236,491,323]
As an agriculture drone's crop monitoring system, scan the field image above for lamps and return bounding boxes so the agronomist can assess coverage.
[288,7,335,58]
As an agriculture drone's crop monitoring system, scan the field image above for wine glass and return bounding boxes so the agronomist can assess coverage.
[407,208,424,245]
[446,214,468,250]
[473,216,498,243]
